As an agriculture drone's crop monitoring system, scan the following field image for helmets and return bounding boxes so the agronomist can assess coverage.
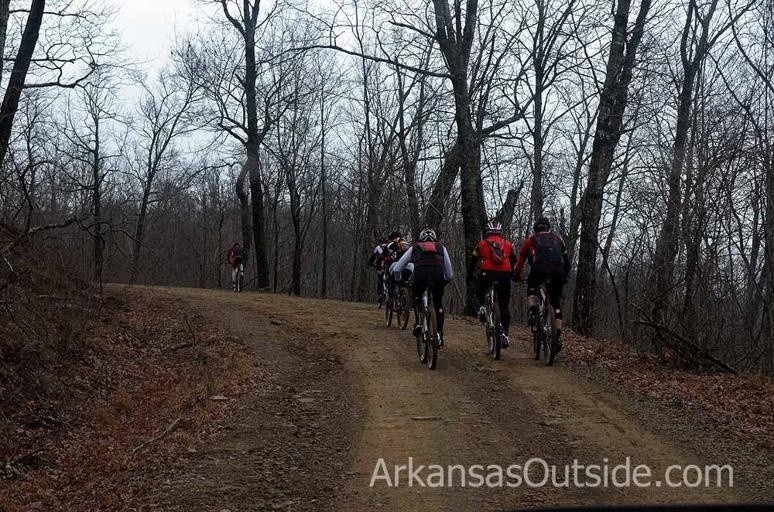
[375,231,401,244]
[418,228,437,242]
[533,216,551,233]
[485,218,503,234]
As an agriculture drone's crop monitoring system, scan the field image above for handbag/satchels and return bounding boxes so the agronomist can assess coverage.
[485,237,507,265]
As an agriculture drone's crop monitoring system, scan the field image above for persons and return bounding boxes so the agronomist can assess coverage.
[377,232,413,303]
[470,220,517,349]
[394,227,453,349]
[227,242,247,287]
[514,218,571,353]
[367,237,389,302]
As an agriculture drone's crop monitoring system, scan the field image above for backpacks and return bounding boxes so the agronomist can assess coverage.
[395,244,411,262]
[412,240,444,267]
[530,233,567,278]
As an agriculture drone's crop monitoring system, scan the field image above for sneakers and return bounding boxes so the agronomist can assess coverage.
[479,306,510,349]
[412,324,445,352]
[376,295,385,303]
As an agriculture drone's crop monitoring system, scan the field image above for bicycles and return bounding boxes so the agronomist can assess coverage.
[368,261,571,369]
[227,256,247,292]
